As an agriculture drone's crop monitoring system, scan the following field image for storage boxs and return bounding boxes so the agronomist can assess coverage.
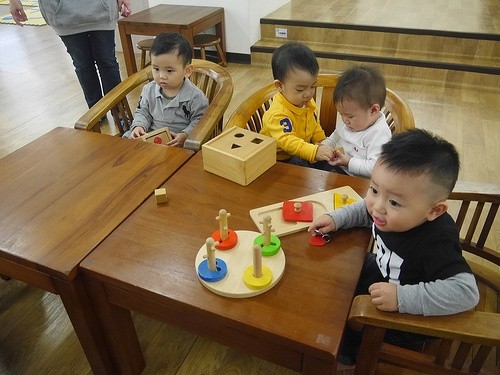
[202,126,276,186]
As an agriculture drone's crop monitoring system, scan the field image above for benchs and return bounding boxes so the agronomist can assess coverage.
[223,73,415,135]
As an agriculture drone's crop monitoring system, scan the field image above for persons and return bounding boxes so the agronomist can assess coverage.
[321,64,393,179]
[308,127,480,370]
[123,32,209,147]
[260,42,334,169]
[9,0,129,124]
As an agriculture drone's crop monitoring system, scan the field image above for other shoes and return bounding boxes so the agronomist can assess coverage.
[114,117,130,128]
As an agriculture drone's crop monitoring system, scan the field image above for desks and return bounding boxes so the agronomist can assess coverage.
[117,3,226,77]
[0,126,372,375]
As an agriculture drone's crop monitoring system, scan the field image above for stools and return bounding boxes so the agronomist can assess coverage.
[194,34,227,67]
[136,39,154,70]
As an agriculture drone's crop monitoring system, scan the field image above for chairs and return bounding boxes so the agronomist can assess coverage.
[74,57,234,150]
[346,181,500,375]
